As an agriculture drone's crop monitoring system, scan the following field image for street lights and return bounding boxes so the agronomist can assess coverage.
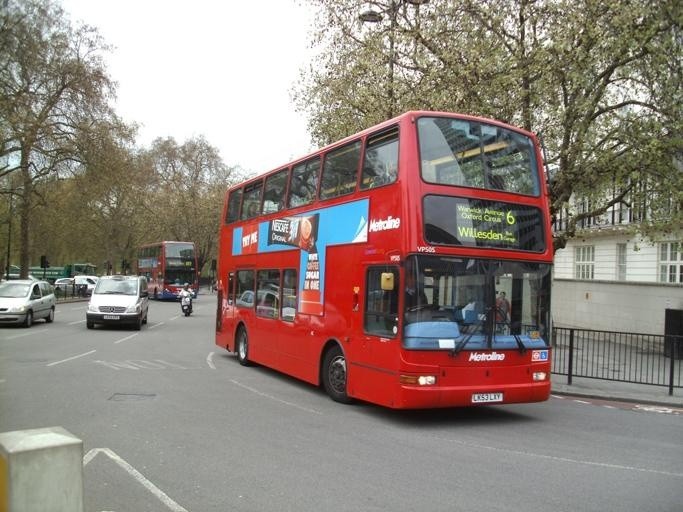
[358,0,430,120]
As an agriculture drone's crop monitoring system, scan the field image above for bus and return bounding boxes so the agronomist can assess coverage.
[215,110,554,409]
[136,241,199,300]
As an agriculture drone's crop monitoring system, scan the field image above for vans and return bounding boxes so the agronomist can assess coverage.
[85,274,149,331]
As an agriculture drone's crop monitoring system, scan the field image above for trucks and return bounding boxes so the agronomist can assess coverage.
[5,262,96,285]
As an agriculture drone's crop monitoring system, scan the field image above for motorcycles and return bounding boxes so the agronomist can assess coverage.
[177,290,194,317]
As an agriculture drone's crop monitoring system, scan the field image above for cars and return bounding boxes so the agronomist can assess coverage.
[0,275,98,329]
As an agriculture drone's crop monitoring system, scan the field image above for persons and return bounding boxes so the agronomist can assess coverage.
[385,270,428,331]
[178,282,196,314]
[245,201,258,217]
[494,291,511,332]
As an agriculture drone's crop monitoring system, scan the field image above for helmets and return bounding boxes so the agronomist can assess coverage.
[184,283,189,290]
